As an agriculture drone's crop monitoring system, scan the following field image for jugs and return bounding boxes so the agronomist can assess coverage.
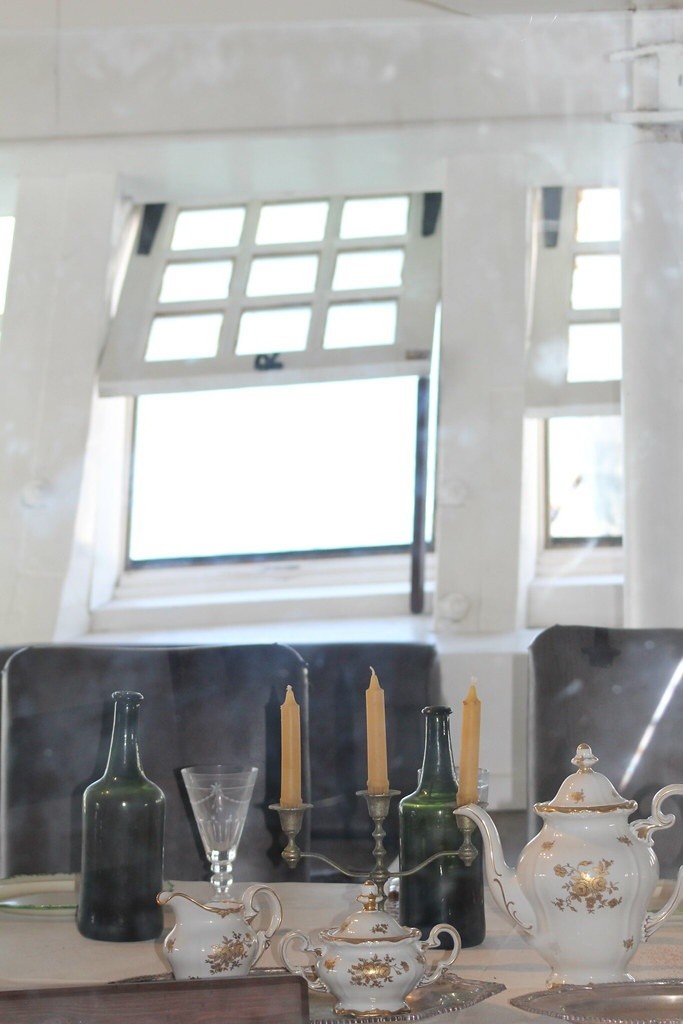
[157,884,282,980]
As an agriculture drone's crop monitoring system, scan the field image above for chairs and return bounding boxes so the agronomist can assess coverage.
[0,642,444,882]
[528,622,683,879]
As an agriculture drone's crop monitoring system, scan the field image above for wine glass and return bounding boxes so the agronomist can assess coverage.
[180,763,258,905]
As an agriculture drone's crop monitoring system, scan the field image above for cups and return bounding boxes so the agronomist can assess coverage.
[279,882,462,1018]
[417,766,491,816]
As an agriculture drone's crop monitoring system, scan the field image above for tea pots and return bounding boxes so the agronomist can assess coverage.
[452,744,683,986]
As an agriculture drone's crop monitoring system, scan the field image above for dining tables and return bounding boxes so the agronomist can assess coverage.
[0,873,683,1024]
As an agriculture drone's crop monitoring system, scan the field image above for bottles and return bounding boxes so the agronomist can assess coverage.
[398,706,485,951]
[75,690,166,942]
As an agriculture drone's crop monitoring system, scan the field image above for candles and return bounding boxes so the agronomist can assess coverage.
[366,666,389,797]
[458,684,481,806]
[277,685,302,810]
